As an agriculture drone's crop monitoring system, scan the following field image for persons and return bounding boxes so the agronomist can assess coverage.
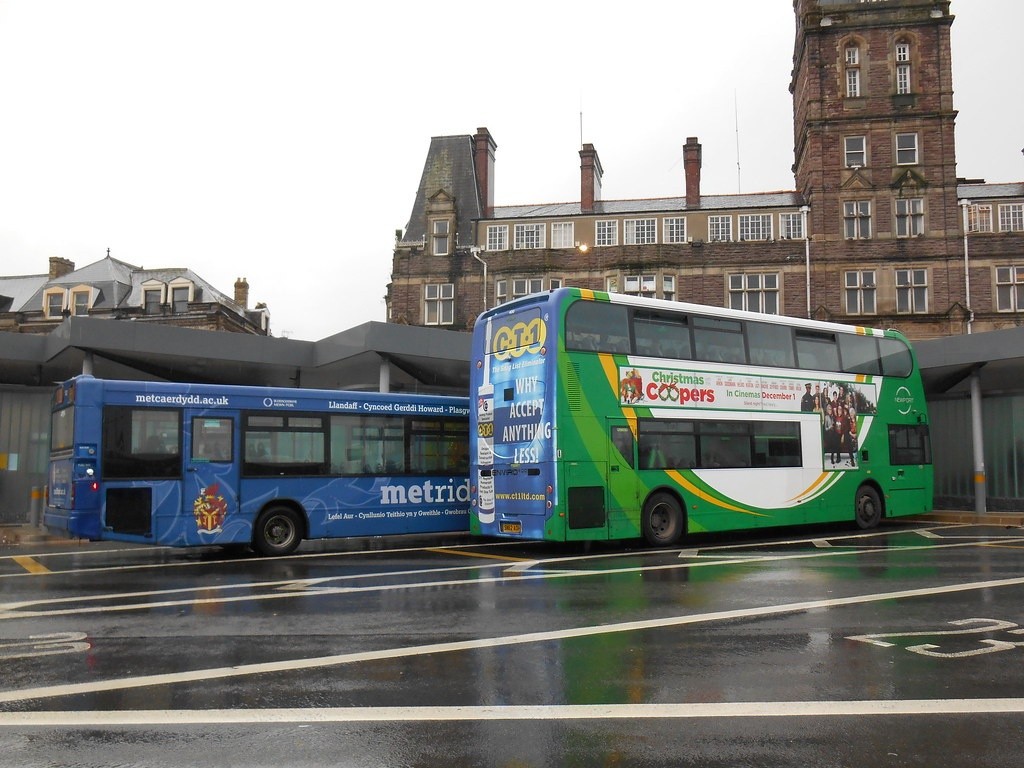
[800,382,866,467]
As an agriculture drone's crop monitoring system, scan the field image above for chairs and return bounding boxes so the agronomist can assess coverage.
[202,442,230,460]
[568,335,778,367]
[246,443,272,462]
[384,459,398,472]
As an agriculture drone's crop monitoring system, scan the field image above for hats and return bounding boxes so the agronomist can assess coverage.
[805,383,812,388]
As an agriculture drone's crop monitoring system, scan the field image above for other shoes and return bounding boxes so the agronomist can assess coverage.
[831,459,834,464]
[851,461,856,467]
[836,458,841,463]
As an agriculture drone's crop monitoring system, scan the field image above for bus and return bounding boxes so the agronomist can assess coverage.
[468,285,934,549]
[43,373,471,557]
